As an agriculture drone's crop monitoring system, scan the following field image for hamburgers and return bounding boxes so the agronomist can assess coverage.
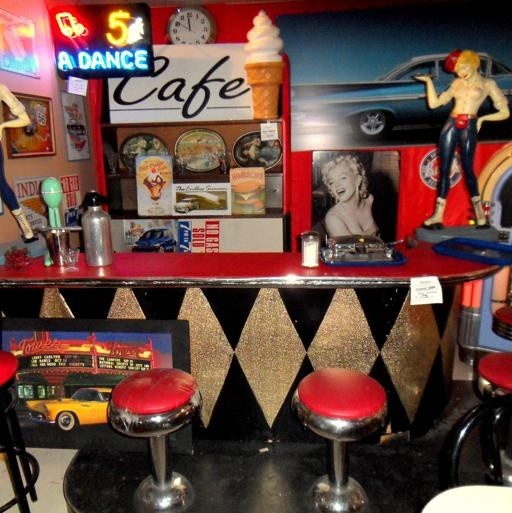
[230,179,265,210]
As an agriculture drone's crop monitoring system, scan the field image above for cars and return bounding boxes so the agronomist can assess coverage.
[23,387,112,435]
[174,197,201,215]
[130,227,177,254]
[292,52,512,142]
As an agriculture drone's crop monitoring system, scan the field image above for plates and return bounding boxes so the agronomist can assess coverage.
[173,128,229,175]
[231,128,285,174]
[118,132,172,178]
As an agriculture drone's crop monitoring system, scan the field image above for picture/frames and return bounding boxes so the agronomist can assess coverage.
[60,89,91,162]
[3,92,57,159]
[1,8,40,79]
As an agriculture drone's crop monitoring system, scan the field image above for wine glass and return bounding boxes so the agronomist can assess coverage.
[60,249,80,272]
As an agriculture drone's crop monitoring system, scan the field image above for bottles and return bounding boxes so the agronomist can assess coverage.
[80,189,115,267]
[301,231,320,267]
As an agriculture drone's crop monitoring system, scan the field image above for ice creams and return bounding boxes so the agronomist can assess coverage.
[243,9,284,119]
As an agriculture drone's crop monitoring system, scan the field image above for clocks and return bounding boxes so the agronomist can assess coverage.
[166,4,216,45]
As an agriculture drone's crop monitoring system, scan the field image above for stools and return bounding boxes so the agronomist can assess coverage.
[1,350,38,507]
[106,368,201,513]
[291,367,389,510]
[440,349,512,485]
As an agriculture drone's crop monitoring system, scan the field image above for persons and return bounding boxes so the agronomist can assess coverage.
[410,44,510,227]
[319,152,381,238]
[0,82,43,240]
